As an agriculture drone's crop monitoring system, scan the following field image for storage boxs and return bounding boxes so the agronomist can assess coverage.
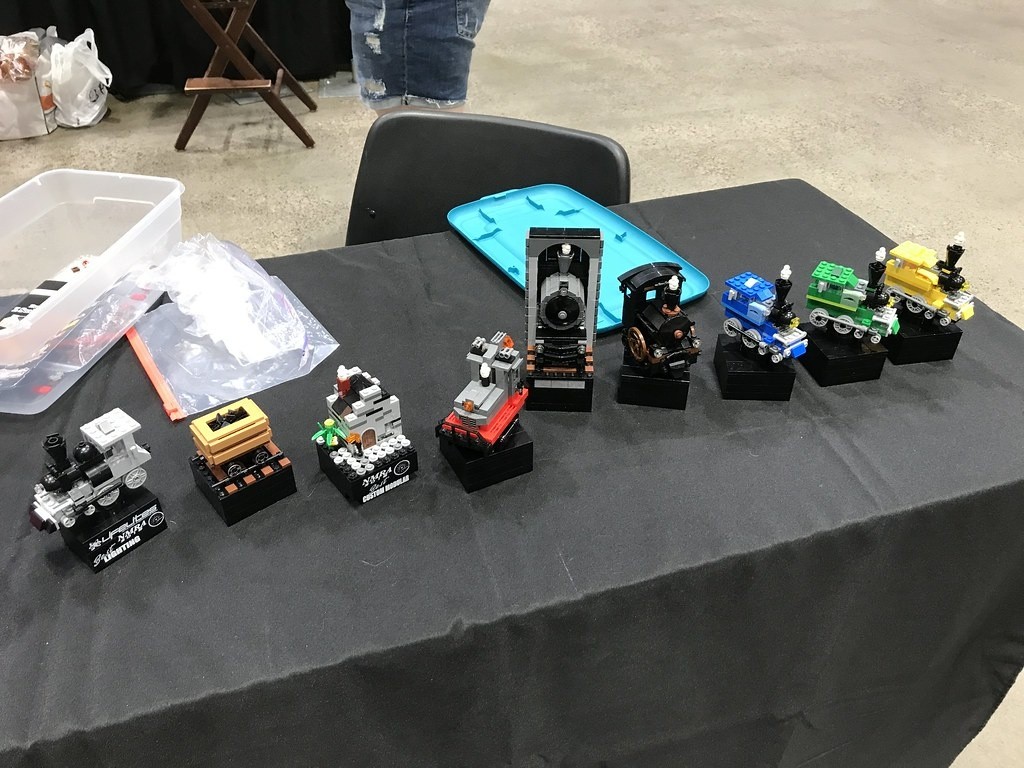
[0,166,186,415]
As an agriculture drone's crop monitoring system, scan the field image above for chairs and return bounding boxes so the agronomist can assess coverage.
[342,111,634,249]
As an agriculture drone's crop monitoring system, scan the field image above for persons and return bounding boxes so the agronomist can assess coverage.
[345,0,491,117]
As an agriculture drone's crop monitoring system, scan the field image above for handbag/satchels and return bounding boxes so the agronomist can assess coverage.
[50,28,112,128]
[0,26,66,140]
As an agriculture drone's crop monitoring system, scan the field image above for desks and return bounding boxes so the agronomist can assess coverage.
[0,177,1024,768]
